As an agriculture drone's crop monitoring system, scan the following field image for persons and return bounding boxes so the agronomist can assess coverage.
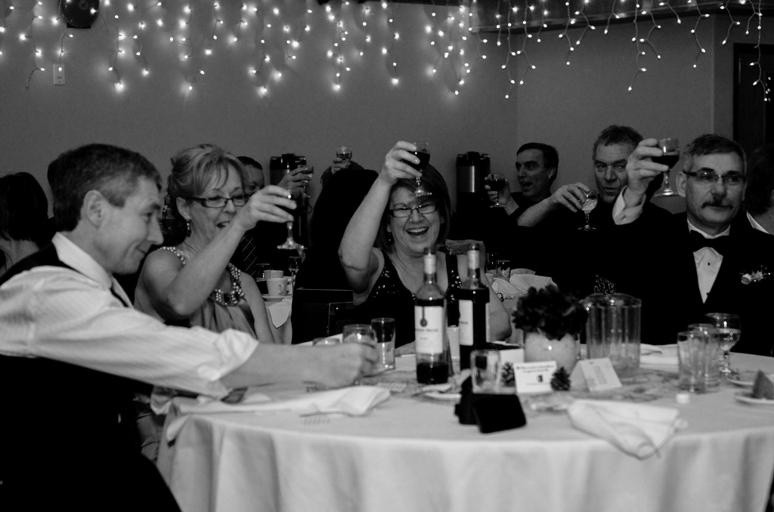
[1,170,51,285]
[591,135,772,358]
[0,142,381,508]
[130,124,674,468]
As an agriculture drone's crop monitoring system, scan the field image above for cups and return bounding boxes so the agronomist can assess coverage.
[312,335,344,350]
[497,260,512,280]
[371,314,399,372]
[341,323,376,382]
[578,290,643,378]
[678,331,709,391]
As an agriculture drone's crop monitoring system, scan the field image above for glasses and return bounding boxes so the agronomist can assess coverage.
[389,203,436,217]
[191,194,246,207]
[685,170,744,184]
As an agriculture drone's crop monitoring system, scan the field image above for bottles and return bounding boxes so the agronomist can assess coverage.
[412,245,448,384]
[456,243,491,373]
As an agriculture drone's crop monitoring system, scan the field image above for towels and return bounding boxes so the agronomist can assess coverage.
[148,383,392,445]
[569,399,684,460]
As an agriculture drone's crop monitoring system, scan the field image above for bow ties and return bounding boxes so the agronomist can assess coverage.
[689,229,728,255]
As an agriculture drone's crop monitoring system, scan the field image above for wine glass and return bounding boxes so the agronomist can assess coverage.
[484,171,508,211]
[675,307,745,393]
[573,186,603,233]
[268,151,317,251]
[335,144,354,162]
[650,133,680,202]
[406,139,436,198]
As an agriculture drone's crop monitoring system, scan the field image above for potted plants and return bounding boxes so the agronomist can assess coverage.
[511,282,582,373]
